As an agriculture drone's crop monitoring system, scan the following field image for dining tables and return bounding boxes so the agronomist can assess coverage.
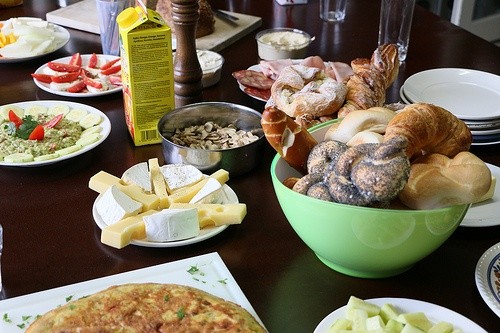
[0,0,500,333]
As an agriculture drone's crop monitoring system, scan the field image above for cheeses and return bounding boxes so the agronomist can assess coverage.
[88,158,246,249]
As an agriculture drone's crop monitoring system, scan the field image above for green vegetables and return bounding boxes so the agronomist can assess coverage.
[4,115,39,140]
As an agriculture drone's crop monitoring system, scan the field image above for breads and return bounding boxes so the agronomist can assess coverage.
[131,0,215,38]
[261,42,492,211]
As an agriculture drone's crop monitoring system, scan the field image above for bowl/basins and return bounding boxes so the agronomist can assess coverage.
[270,119,472,279]
[157,102,265,177]
[253,27,312,62]
[195,49,225,88]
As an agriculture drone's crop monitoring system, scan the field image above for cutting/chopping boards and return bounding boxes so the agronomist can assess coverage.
[46,0,264,51]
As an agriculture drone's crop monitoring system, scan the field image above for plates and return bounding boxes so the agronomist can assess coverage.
[237,64,268,103]
[34,54,122,97]
[460,162,500,227]
[474,242,500,317]
[313,298,488,333]
[92,173,241,248]
[0,100,112,167]
[399,68,500,145]
[0,250,271,332]
[0,24,71,63]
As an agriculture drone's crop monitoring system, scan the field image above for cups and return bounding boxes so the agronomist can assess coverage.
[97,0,129,56]
[320,0,346,22]
[378,0,416,60]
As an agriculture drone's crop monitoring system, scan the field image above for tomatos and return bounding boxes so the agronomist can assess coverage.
[45,114,63,128]
[28,125,44,140]
[8,110,23,127]
[30,51,121,92]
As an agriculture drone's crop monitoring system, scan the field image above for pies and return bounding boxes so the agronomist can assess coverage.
[24,283,268,333]
